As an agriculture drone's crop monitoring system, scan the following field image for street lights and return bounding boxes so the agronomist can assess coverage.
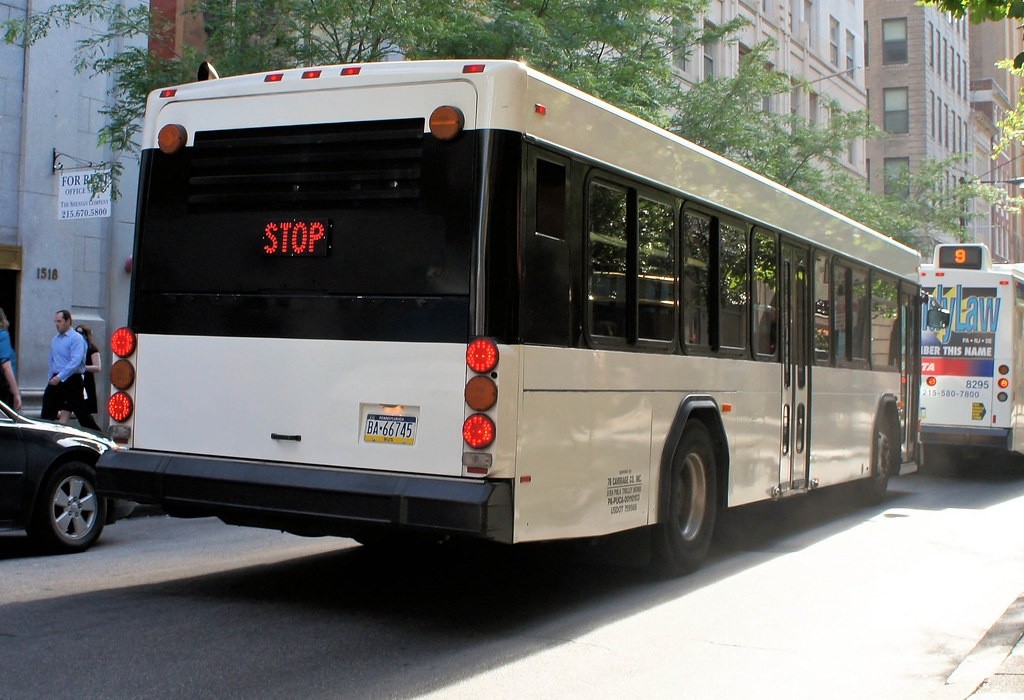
[955,172,1024,243]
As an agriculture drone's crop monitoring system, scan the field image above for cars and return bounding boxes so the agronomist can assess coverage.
[0,395,120,552]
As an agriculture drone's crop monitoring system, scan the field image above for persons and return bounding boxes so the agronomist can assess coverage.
[0,308,22,419]
[40,307,103,432]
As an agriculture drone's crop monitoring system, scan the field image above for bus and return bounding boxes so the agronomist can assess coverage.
[93,62,929,580]
[916,243,1024,467]
